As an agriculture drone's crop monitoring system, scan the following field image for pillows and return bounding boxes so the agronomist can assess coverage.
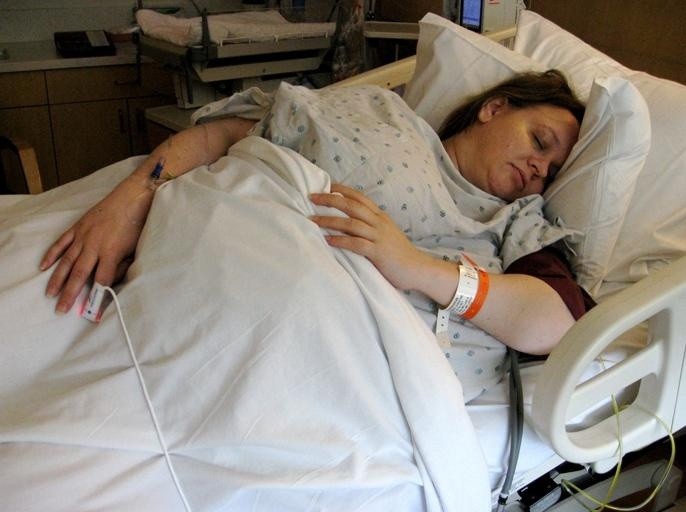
[402,12,651,301]
[509,10,686,283]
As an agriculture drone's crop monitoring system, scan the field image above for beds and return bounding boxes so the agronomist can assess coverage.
[0,25,686,512]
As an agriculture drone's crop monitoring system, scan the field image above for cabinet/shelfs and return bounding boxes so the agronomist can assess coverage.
[0,40,171,192]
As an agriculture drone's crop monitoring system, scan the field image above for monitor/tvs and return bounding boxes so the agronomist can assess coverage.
[458,0,517,36]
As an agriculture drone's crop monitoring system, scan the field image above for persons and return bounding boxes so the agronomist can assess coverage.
[35,68,589,365]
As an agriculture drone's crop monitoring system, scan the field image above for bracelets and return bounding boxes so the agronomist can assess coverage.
[458,268,490,321]
[439,264,479,316]
